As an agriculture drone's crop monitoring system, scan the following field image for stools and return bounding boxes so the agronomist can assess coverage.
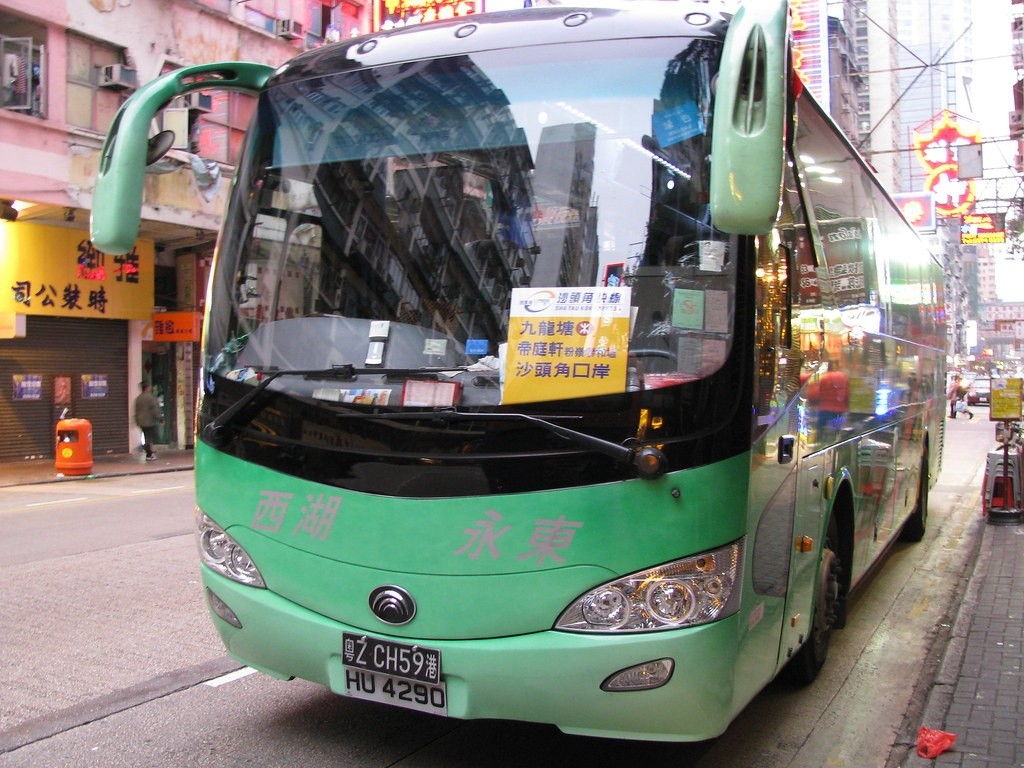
[980,448,1023,518]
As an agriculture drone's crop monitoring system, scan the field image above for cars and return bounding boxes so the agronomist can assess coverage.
[964,372,990,406]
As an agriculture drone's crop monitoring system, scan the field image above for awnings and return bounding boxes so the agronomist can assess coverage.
[1,174,226,258]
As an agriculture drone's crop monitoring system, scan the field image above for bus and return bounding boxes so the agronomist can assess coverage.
[89,0,949,743]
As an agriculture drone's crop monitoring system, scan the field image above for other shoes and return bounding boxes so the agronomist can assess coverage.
[146,453,157,460]
[969,413,973,419]
[948,415,956,418]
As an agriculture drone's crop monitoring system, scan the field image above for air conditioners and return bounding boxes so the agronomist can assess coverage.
[181,92,213,113]
[100,63,136,89]
[278,19,305,39]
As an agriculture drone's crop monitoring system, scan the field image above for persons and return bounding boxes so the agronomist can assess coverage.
[135,380,164,460]
[947,374,974,419]
[815,361,849,442]
[909,372,932,395]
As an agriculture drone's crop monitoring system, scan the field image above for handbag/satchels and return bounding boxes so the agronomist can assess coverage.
[955,401,967,413]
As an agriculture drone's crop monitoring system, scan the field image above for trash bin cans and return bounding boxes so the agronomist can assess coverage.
[54,418,94,475]
